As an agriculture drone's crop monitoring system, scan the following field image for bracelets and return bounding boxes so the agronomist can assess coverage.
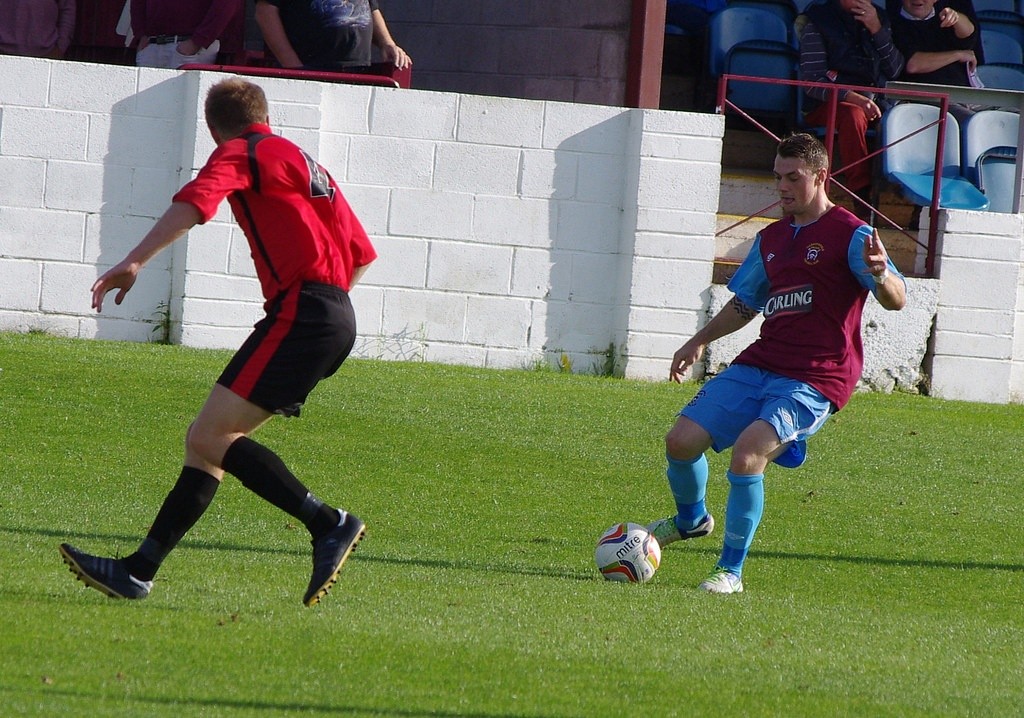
[872,269,889,284]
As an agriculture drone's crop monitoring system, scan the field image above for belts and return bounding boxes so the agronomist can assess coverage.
[150,35,190,44]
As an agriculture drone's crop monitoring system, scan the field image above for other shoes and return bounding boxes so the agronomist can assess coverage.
[855,182,877,228]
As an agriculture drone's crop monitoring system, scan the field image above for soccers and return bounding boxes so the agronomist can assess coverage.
[595,521,662,584]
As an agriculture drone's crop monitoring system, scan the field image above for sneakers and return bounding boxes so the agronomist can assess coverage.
[304,508,366,608]
[648,512,712,544]
[703,565,746,594]
[59,543,153,602]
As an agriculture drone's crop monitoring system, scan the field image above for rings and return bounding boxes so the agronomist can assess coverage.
[862,11,866,15]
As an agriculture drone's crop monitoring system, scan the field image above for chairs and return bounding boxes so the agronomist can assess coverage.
[705,0,1024,210]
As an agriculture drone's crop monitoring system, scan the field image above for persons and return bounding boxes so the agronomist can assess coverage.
[0,0,412,84]
[58,77,366,607]
[645,132,905,597]
[798,0,984,230]
